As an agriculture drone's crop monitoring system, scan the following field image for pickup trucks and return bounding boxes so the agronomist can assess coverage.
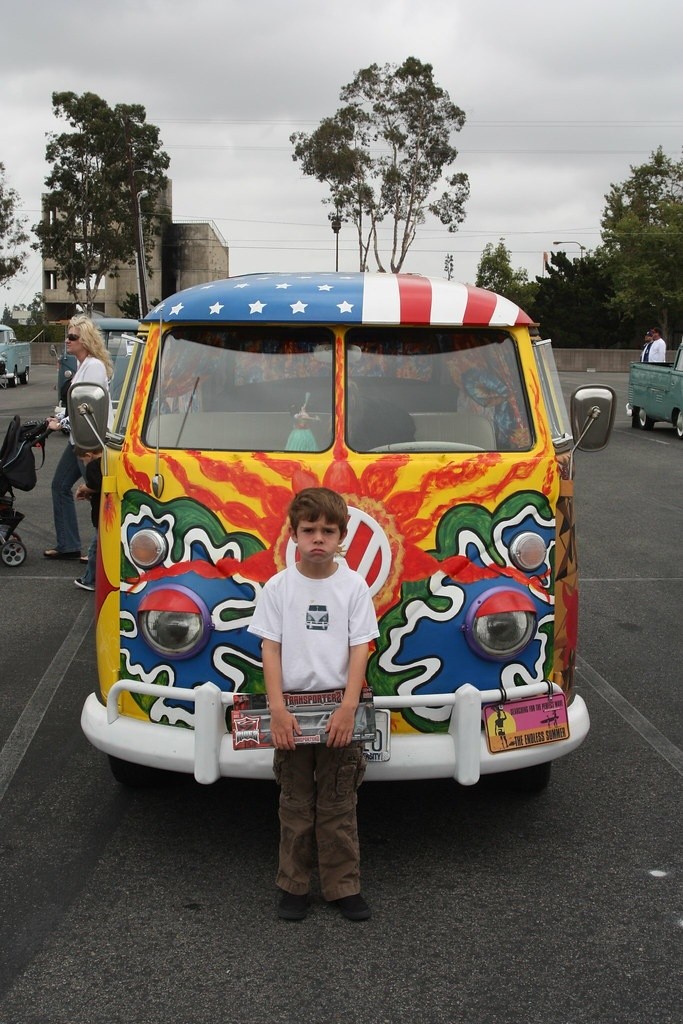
[0,324,31,387]
[627,337,683,439]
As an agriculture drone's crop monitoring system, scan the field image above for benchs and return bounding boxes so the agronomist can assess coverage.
[147,373,498,452]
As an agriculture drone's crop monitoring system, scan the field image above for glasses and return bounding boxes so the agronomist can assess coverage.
[651,331,657,333]
[66,333,81,340]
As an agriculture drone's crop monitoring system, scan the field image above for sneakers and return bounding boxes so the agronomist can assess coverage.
[336,894,371,921]
[625,402,632,416]
[73,577,95,590]
[276,888,312,920]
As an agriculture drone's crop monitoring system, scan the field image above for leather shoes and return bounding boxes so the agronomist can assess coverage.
[42,546,80,559]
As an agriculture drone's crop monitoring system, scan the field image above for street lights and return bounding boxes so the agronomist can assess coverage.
[553,240,584,260]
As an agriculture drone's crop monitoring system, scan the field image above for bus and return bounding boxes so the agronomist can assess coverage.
[51,272,618,806]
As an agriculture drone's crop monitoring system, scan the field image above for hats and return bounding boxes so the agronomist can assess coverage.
[645,330,652,336]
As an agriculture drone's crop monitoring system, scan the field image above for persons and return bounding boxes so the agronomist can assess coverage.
[41,312,112,565]
[246,485,378,923]
[648,327,666,363]
[72,443,105,590]
[639,331,654,361]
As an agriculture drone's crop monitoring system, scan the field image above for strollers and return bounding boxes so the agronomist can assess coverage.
[0,414,57,566]
